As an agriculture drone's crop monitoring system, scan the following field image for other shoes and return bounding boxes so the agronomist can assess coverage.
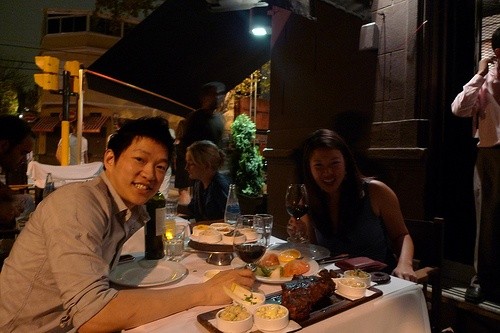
[465,286,495,302]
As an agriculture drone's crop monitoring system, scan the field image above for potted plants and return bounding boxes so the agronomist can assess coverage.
[228,114,269,228]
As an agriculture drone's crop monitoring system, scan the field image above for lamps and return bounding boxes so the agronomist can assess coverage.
[250,6,270,35]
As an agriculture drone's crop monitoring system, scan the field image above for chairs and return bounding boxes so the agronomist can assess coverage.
[404,216,446,333]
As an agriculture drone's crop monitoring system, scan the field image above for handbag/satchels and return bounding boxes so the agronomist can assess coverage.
[335,256,388,272]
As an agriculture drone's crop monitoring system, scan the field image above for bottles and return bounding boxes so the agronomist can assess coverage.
[225,184,241,228]
[144,189,166,260]
[43,172,54,197]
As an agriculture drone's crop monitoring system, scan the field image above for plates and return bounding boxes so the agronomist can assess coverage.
[231,251,319,283]
[110,259,187,287]
[268,242,330,260]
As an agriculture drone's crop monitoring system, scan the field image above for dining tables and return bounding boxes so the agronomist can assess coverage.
[115,235,435,333]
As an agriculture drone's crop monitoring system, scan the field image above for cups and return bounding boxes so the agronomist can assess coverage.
[253,214,273,249]
[163,203,186,261]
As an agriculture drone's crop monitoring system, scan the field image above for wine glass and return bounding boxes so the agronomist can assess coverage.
[231,215,267,272]
[286,184,310,243]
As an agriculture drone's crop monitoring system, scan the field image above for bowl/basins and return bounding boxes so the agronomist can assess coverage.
[254,304,288,330]
[344,270,371,287]
[337,278,367,298]
[234,292,266,317]
[216,308,253,333]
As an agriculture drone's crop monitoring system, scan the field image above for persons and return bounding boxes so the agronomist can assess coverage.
[287,129,418,284]
[175,140,234,222]
[450,27,500,304]
[56,120,88,165]
[174,82,226,189]
[0,113,256,333]
